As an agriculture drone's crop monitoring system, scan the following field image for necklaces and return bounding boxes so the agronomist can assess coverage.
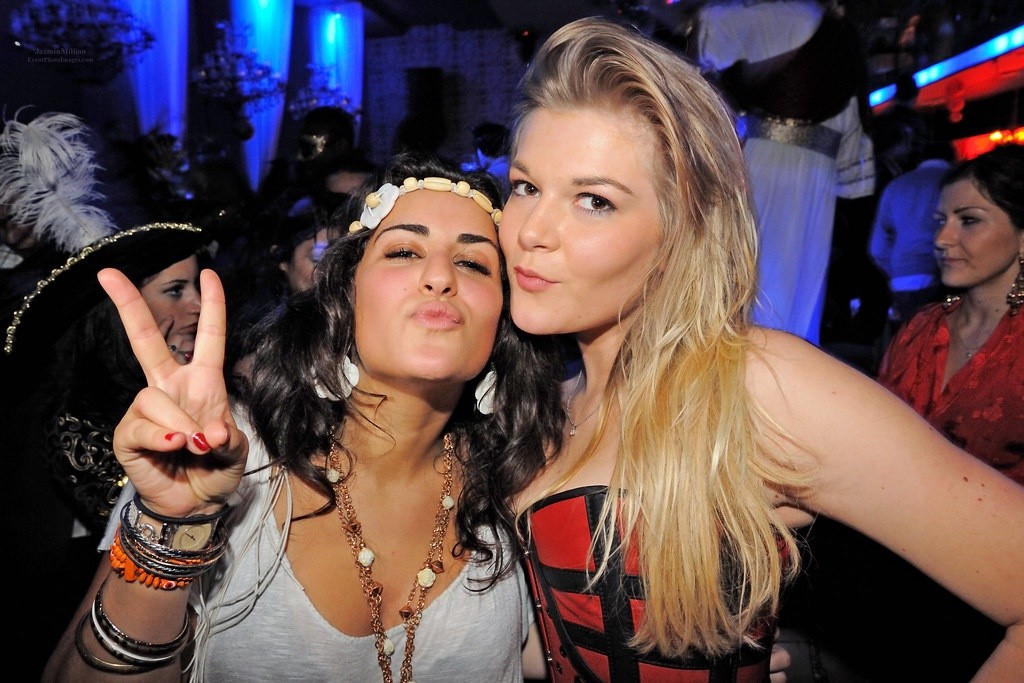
[321,426,455,683]
[955,319,985,359]
[566,370,599,437]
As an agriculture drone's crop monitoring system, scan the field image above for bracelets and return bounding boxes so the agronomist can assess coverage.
[74,580,190,675]
[110,492,230,591]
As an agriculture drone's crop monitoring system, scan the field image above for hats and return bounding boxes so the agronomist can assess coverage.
[0,103,205,356]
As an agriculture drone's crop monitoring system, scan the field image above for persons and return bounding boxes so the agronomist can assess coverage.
[455,17,1024,683]
[42,153,584,683]
[761,142,1024,532]
[0,105,383,553]
[868,133,958,335]
